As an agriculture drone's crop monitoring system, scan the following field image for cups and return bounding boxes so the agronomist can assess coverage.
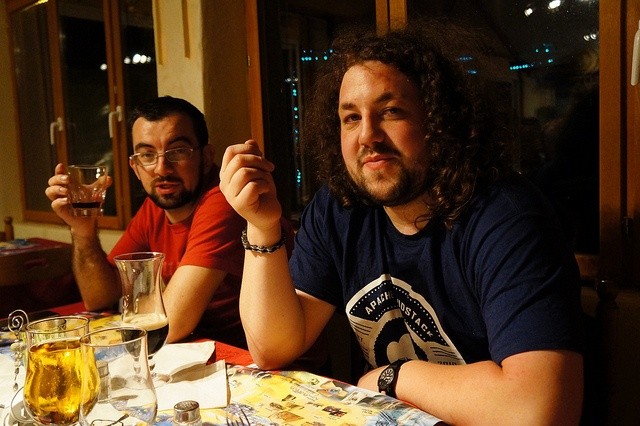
[65,164,109,216]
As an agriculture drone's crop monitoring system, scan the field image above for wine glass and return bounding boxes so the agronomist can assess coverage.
[23,315,99,426]
[114,251,169,391]
[78,326,158,425]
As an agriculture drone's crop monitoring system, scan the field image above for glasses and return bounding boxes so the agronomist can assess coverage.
[129,148,196,167]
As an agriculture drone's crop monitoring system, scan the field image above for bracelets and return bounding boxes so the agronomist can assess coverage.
[240,232,290,254]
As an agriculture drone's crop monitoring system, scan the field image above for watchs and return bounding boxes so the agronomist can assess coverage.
[377,356,410,398]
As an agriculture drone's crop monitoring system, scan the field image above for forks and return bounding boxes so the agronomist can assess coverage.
[226,409,252,426]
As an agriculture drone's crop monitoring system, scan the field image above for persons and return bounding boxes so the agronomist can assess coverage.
[45,96,247,345]
[217,35,585,426]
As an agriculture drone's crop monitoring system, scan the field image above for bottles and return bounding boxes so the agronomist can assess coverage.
[172,400,203,426]
